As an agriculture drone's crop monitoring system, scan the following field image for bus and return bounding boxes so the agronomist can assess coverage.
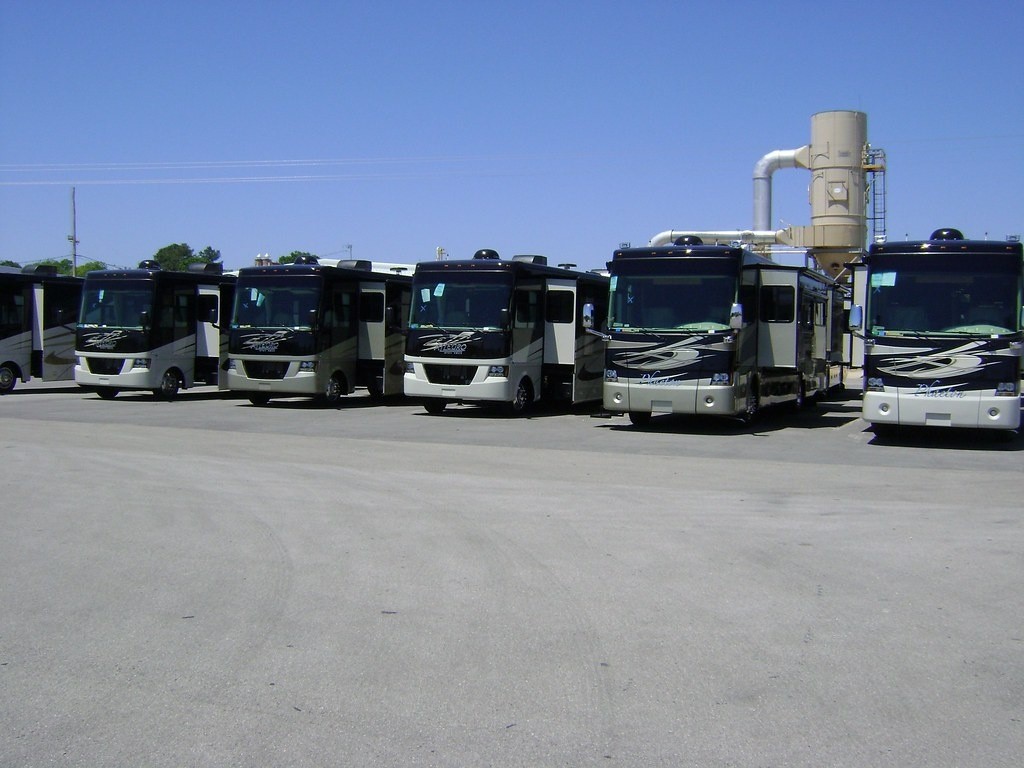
[0,263,92,394]
[845,228,1024,437]
[56,259,261,402]
[205,251,445,407]
[582,234,850,429]
[385,248,628,414]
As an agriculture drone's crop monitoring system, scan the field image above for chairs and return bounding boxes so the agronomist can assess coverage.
[972,306,1004,326]
[895,305,928,330]
[272,314,294,327]
[644,306,672,328]
[712,306,736,323]
[447,311,467,326]
